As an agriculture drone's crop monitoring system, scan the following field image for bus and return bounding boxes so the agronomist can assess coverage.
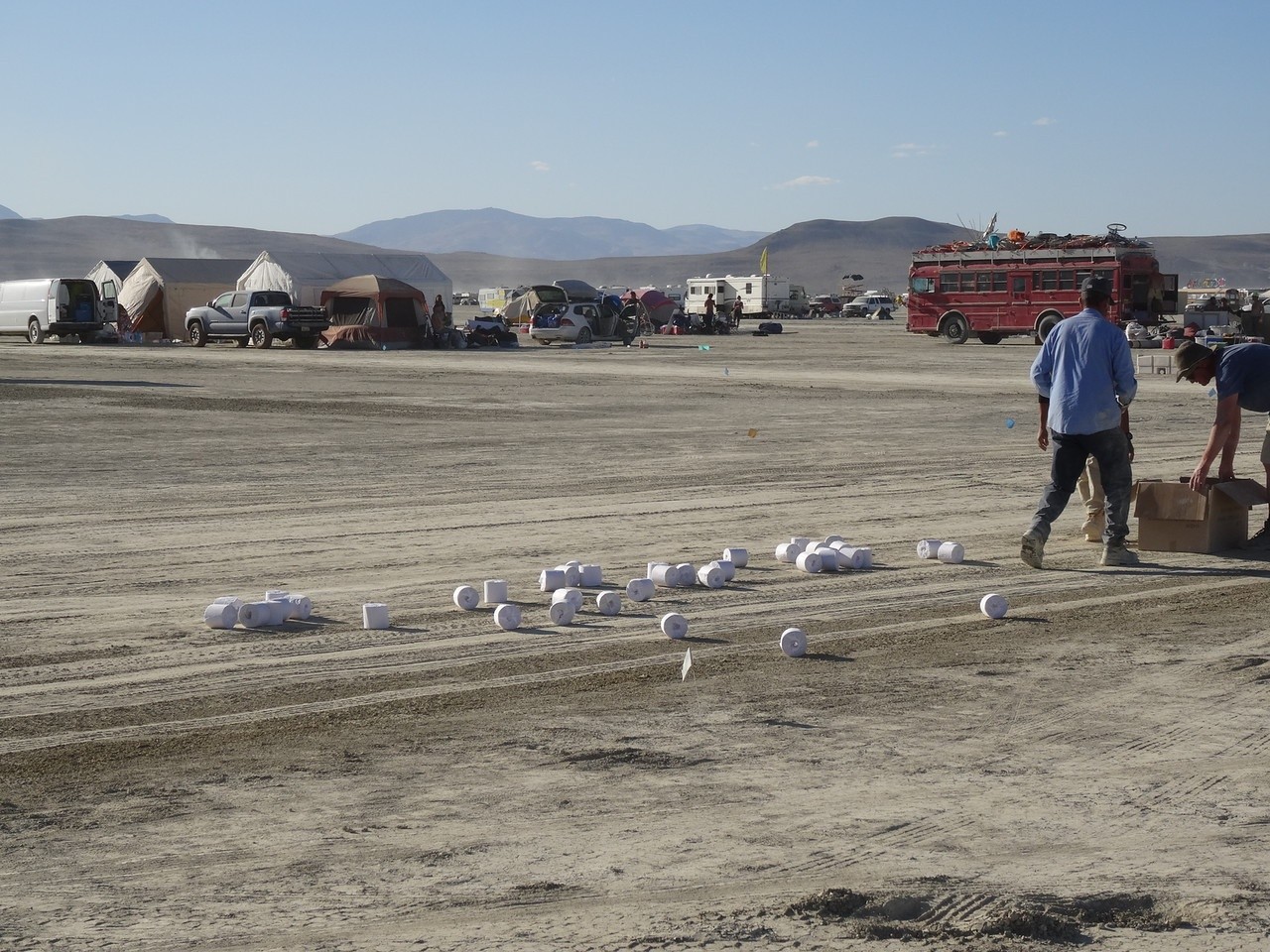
[1176,288,1248,304]
[904,234,1179,347]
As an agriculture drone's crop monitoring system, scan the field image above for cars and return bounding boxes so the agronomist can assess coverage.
[1242,298,1270,314]
[1185,298,1222,311]
[811,293,843,316]
[452,294,477,306]
[526,284,642,345]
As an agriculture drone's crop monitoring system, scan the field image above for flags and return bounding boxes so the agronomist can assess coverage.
[760,247,769,274]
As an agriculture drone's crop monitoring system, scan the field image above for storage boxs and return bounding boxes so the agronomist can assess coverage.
[142,332,163,344]
[1130,477,1267,554]
[1137,354,1177,375]
[660,325,683,335]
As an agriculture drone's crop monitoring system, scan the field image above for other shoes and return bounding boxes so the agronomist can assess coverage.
[1081,508,1108,534]
[1084,531,1102,543]
[1239,520,1270,551]
[1020,530,1045,571]
[1099,545,1139,566]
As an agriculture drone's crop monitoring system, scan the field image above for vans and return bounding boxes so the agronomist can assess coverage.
[0,277,120,346]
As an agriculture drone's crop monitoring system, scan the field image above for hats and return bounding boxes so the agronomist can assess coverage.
[1081,276,1116,306]
[1175,341,1217,383]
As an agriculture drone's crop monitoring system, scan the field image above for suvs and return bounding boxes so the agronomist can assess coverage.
[842,295,894,318]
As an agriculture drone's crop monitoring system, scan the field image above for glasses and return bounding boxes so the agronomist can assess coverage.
[1185,366,1200,381]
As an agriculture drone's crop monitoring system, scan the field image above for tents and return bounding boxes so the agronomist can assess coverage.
[620,288,680,335]
[83,247,454,352]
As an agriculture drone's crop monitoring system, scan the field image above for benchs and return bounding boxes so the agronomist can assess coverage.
[1205,336,1264,348]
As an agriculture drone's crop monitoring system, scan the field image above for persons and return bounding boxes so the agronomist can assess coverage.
[619,291,647,348]
[704,293,717,317]
[1203,291,1265,338]
[1174,340,1270,548]
[1020,272,1138,566]
[1077,401,1136,541]
[429,295,445,339]
[733,295,744,329]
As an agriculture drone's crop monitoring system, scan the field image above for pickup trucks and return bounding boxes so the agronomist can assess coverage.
[184,289,332,350]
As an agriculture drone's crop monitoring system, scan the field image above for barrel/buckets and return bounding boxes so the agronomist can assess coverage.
[1162,337,1174,349]
[74,294,91,322]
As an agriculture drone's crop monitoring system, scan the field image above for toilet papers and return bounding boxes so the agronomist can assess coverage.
[626,578,655,602]
[493,604,521,630]
[362,602,390,630]
[539,560,603,592]
[916,539,965,564]
[780,627,808,658]
[775,534,873,574]
[204,589,313,629]
[483,579,508,603]
[452,585,480,610]
[660,612,687,639]
[646,547,748,588]
[596,589,622,616]
[980,593,1008,619]
[549,587,584,625]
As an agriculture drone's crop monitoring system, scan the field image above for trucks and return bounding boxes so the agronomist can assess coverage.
[685,274,810,320]
